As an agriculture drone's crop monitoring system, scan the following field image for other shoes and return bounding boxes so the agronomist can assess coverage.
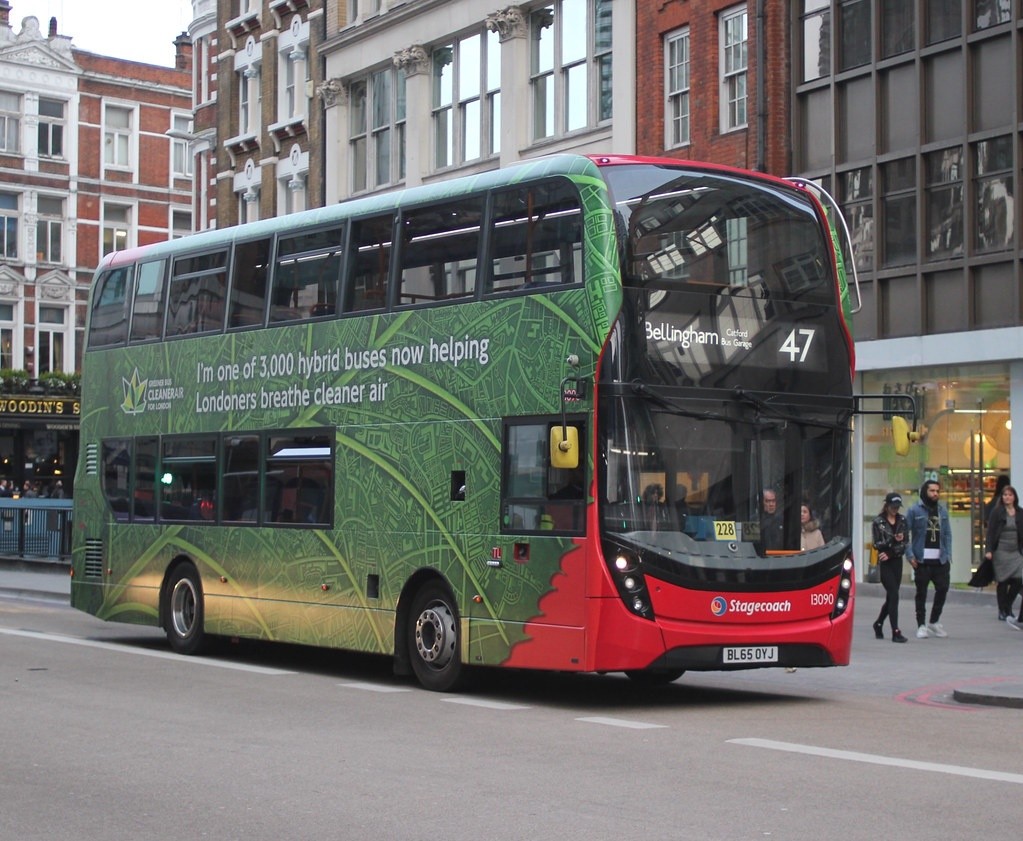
[873,621,907,643]
[999,609,1015,620]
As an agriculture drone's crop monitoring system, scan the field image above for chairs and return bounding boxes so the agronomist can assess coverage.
[542,504,575,531]
[167,305,359,336]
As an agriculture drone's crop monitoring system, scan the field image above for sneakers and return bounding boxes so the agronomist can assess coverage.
[1006,616,1023,631]
[915,623,946,638]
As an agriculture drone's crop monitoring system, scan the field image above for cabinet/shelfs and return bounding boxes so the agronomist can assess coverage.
[924,467,1010,564]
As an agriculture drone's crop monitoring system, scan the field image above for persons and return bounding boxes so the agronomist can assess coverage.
[0,473,69,500]
[967,486,1023,619]
[904,478,951,637]
[983,475,1010,526]
[609,479,738,535]
[751,488,784,553]
[799,505,825,551]
[1006,592,1023,632]
[871,492,909,643]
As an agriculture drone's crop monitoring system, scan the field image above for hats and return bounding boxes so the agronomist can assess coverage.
[886,493,904,507]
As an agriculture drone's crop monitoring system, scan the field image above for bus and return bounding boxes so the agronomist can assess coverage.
[69,154,861,691]
